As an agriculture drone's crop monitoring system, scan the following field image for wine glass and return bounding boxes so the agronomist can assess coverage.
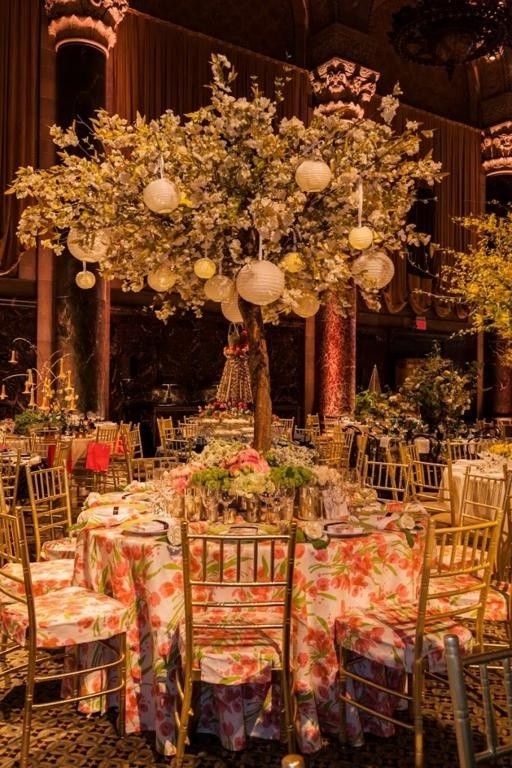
[202,484,295,527]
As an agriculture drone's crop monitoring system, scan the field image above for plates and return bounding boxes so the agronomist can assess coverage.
[321,529,368,537]
[218,529,271,542]
[93,504,174,535]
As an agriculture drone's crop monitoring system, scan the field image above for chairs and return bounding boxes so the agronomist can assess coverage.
[443,632,511,768]
[335,510,504,766]
[0,415,512,603]
[0,506,130,768]
[175,521,297,767]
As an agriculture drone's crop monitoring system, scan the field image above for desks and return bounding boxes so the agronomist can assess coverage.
[61,479,429,753]
